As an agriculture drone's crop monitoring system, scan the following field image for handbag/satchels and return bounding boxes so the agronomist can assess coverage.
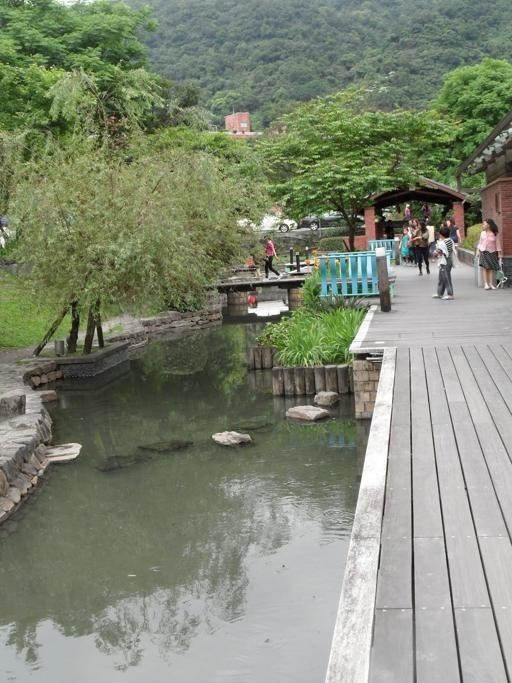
[449,249,460,267]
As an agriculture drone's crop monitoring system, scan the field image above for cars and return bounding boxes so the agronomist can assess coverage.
[238,213,296,233]
[294,211,366,230]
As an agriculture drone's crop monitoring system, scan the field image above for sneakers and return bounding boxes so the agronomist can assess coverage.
[485,283,496,290]
[433,294,453,300]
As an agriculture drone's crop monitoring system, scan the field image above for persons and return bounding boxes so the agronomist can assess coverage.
[474,218,502,290]
[430,227,457,301]
[263,235,282,278]
[399,203,462,275]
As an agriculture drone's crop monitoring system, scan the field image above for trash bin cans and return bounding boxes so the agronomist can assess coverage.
[55,341,64,357]
